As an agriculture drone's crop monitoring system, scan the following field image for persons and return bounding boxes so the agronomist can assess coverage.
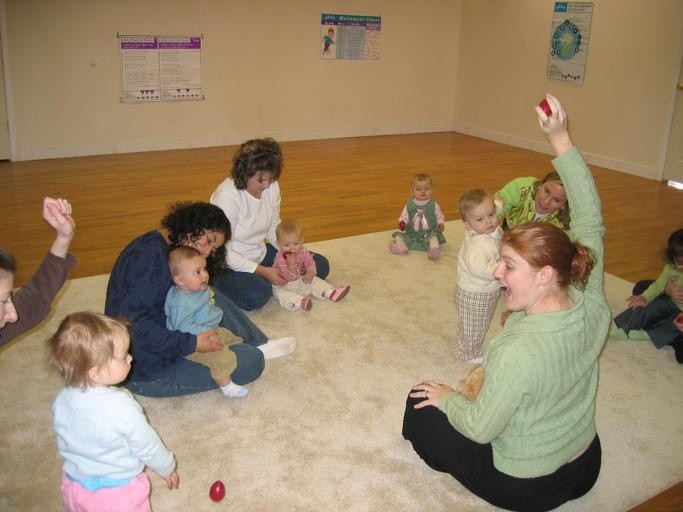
[452,189,506,368]
[163,244,250,398]
[268,218,351,310]
[206,136,329,310]
[100,199,298,398]
[386,172,445,260]
[0,195,78,346]
[486,167,572,232]
[48,310,180,512]
[608,228,683,367]
[397,91,608,511]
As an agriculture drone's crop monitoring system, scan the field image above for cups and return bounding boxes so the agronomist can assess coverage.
[0,295,15,309]
[205,228,218,258]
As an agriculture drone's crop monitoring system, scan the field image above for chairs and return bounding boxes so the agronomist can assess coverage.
[302,298,312,310]
[388,244,410,256]
[330,284,351,302]
[426,247,442,261]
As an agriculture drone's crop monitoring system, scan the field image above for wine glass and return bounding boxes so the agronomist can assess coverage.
[424,390,428,400]
